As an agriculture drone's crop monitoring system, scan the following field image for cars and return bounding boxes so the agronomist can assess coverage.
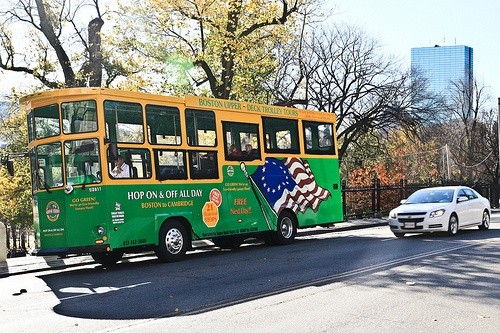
[387,184,491,238]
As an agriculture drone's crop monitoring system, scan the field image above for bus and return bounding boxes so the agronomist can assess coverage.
[17,85,347,264]
